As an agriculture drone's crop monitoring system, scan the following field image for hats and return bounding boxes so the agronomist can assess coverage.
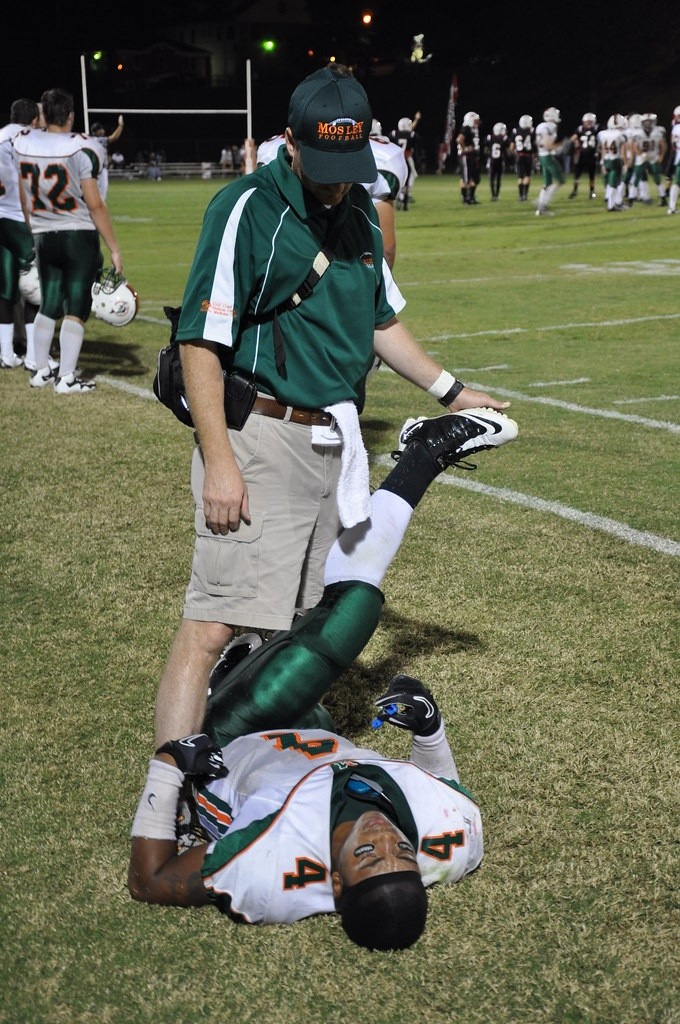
[288,65,378,183]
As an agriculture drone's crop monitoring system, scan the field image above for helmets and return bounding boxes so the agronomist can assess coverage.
[17,250,43,306]
[91,267,139,327]
[395,108,680,134]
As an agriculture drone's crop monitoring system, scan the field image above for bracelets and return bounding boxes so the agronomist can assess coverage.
[437,381,465,406]
[426,368,458,400]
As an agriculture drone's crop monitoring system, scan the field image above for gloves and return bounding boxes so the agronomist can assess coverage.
[154,732,230,780]
[377,672,440,738]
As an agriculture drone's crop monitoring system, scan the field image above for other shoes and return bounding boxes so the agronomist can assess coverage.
[395,190,680,215]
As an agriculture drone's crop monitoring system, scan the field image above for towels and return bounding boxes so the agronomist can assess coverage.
[311,399,373,530]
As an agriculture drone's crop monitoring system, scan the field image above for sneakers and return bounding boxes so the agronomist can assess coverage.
[174,792,212,862]
[0,354,24,369]
[30,369,58,389]
[389,408,519,472]
[205,631,263,691]
[54,373,96,394]
[21,358,59,374]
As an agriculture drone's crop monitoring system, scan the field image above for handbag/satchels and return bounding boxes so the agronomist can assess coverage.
[153,305,256,431]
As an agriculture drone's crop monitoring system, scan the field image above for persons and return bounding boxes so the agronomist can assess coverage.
[0,88,680,394]
[128,408,517,953]
[156,62,513,751]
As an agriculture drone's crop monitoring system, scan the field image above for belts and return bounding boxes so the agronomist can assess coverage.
[250,395,337,431]
